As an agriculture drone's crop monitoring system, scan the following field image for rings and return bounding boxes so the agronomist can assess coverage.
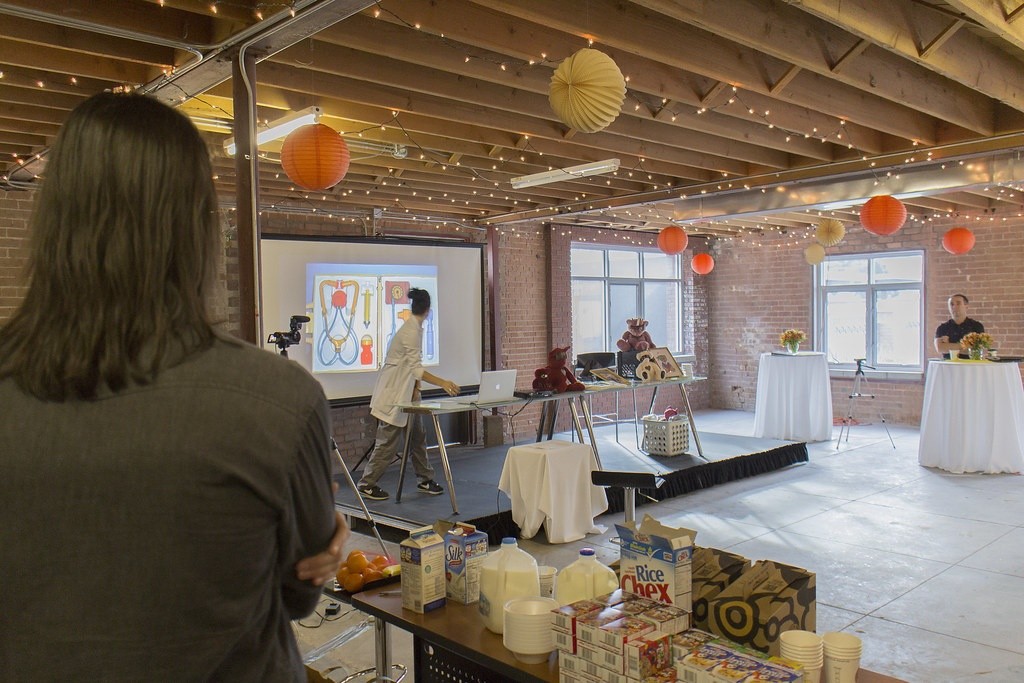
[450,389,452,391]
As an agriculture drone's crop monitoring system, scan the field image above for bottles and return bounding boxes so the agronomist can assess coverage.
[557,548,619,607]
[478,538,540,635]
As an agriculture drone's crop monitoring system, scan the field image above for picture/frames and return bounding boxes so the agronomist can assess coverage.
[649,347,684,378]
[589,368,628,384]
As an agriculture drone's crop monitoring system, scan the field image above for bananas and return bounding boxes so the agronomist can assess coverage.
[382,564,401,578]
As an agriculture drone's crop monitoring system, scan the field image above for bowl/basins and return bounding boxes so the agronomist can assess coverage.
[503,597,559,664]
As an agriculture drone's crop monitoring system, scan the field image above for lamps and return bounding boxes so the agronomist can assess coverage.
[221,105,323,159]
[510,158,621,190]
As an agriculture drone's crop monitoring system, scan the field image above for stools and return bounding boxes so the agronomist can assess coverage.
[590,470,667,547]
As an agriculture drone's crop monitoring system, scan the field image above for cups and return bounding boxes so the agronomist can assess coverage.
[950,350,960,360]
[988,349,998,356]
[538,566,558,598]
[779,630,823,683]
[823,632,864,683]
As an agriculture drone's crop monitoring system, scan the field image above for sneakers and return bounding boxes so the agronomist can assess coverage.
[357,486,388,500]
[417,480,444,494]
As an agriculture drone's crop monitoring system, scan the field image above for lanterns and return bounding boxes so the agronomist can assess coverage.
[657,224,688,254]
[691,252,714,275]
[942,226,975,256]
[280,123,351,190]
[860,194,907,237]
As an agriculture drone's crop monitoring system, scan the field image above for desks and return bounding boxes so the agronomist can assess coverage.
[755,352,833,442]
[547,375,707,471]
[918,361,1024,476]
[396,390,585,517]
[322,531,409,683]
[496,438,610,545]
[350,579,905,683]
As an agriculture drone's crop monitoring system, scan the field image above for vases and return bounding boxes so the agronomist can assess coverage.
[787,342,800,353]
[968,348,985,361]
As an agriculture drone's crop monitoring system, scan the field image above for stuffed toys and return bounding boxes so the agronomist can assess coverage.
[636,350,666,381]
[617,318,656,352]
[545,346,585,392]
[533,367,557,393]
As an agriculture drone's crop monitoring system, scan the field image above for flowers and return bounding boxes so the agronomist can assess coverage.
[781,328,809,347]
[959,332,997,349]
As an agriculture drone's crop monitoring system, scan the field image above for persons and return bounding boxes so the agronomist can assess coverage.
[357,288,459,500]
[0,89,350,683]
[934,293,985,359]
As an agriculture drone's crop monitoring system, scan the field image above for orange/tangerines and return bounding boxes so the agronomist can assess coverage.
[335,550,391,593]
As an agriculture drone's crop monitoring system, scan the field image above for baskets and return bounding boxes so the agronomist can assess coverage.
[640,415,689,457]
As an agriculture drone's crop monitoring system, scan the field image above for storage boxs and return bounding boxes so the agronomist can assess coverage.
[551,514,816,683]
